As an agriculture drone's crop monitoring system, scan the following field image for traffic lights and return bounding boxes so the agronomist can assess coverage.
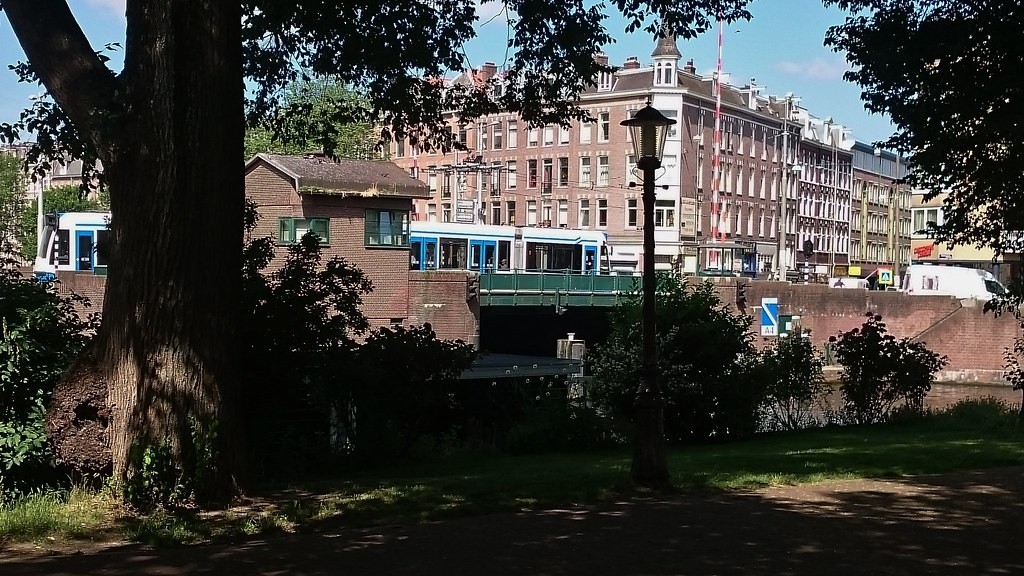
[803,240,814,257]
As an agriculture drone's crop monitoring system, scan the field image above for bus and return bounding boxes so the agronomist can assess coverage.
[35,212,112,280]
[410,219,613,277]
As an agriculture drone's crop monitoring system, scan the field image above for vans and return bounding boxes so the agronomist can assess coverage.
[903,265,1014,303]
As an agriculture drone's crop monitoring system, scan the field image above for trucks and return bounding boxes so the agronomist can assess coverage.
[829,277,870,290]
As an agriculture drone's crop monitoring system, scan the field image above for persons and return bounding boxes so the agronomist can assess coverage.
[498,259,508,271]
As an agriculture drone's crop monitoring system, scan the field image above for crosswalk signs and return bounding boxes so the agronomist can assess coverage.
[882,272,890,282]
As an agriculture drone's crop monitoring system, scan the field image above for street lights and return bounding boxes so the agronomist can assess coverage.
[622,98,677,488]
